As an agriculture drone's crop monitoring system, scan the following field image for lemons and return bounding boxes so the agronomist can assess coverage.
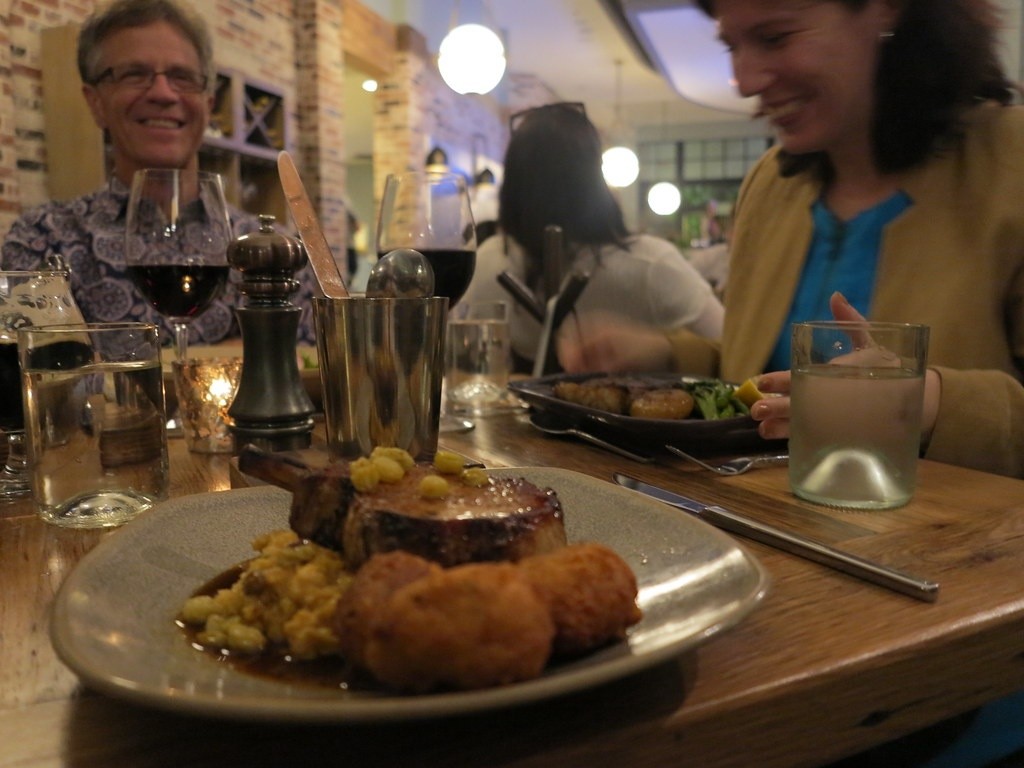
[733,379,764,405]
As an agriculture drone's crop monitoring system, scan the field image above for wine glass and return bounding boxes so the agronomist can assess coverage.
[120,168,231,436]
[0,271,94,503]
[374,170,478,434]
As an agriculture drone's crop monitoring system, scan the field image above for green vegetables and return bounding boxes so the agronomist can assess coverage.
[682,377,750,420]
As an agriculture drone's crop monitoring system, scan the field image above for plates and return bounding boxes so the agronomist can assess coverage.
[47,470,767,723]
[294,363,325,407]
[508,361,786,458]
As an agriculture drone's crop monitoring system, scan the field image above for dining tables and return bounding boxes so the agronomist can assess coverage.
[0,372,1024,768]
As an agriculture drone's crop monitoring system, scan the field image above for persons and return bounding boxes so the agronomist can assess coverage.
[557,0,1024,768]
[0,0,332,417]
[449,103,725,371]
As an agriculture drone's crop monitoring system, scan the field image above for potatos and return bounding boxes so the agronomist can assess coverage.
[554,381,694,419]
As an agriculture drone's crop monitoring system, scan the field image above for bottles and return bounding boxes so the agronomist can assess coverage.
[228,213,314,454]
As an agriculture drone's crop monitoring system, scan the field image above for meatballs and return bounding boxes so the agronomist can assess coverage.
[327,549,443,688]
[379,560,554,688]
[514,540,647,656]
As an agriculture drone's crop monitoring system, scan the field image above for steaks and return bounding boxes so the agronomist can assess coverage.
[289,455,568,573]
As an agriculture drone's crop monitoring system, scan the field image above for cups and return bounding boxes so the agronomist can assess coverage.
[18,318,172,529]
[275,149,350,300]
[448,295,511,410]
[172,357,245,455]
[788,319,931,509]
[312,290,450,466]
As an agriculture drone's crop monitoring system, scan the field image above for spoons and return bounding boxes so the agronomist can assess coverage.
[365,251,435,299]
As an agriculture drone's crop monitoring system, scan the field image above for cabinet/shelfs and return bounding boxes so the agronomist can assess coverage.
[40,24,292,227]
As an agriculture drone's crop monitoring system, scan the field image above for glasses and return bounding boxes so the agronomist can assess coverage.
[508,100,588,135]
[90,60,209,94]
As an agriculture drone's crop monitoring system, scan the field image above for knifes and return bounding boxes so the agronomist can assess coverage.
[610,471,943,603]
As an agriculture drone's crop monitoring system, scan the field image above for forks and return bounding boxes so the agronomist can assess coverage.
[666,441,788,478]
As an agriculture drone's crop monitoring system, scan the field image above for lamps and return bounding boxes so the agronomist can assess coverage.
[602,59,640,187]
[426,107,448,174]
[473,134,497,191]
